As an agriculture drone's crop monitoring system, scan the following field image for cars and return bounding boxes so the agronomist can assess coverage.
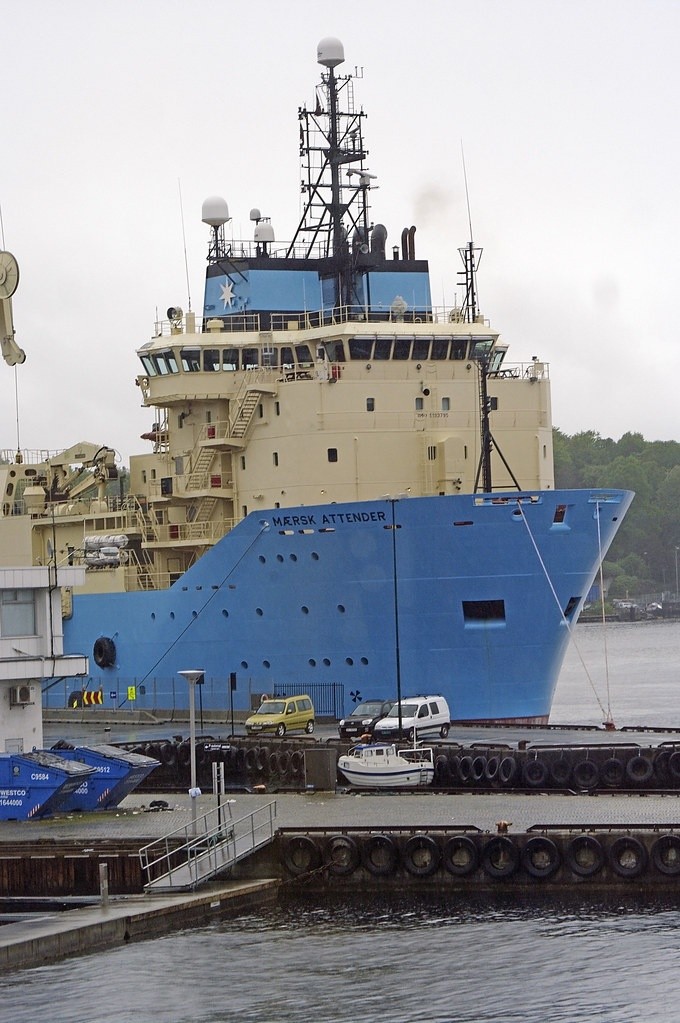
[616,601,637,609]
[644,602,662,610]
[613,598,636,607]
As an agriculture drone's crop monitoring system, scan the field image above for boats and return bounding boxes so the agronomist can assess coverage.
[0,37,636,727]
[338,733,434,785]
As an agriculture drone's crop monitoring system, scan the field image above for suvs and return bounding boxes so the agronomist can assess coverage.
[337,699,395,738]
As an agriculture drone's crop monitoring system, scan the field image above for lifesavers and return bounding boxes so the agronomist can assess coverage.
[361,834,402,878]
[401,835,443,879]
[433,750,680,789]
[480,835,521,881]
[651,834,680,876]
[281,834,322,876]
[321,835,361,878]
[67,690,91,708]
[607,836,650,879]
[520,836,563,881]
[131,743,304,777]
[564,835,606,879]
[442,835,480,878]
[92,636,117,668]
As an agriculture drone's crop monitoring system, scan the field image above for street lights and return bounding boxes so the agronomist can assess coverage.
[177,671,205,836]
[675,546,679,597]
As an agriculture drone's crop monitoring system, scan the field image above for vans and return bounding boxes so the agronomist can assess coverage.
[245,695,316,736]
[374,693,451,741]
